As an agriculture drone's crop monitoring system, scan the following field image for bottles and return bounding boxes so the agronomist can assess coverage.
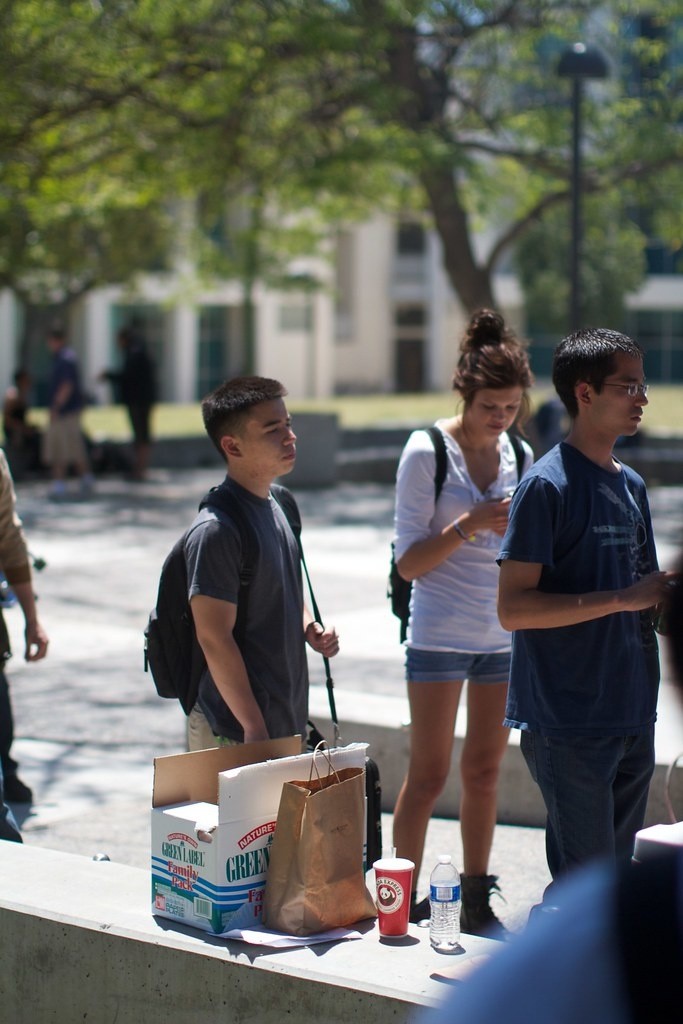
[429,854,461,950]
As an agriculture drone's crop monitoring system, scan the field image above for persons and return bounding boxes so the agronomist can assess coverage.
[393,307,535,941]
[3,368,40,471]
[100,326,161,481]
[0,448,49,840]
[45,322,98,498]
[188,376,339,754]
[423,561,683,1023]
[495,328,683,933]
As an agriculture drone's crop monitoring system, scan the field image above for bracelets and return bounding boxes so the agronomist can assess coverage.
[454,521,476,541]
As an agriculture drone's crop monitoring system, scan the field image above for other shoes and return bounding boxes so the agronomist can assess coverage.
[3,780,31,802]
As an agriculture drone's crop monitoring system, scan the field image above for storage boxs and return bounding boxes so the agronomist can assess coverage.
[151,734,303,934]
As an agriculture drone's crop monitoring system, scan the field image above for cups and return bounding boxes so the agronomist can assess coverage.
[373,857,415,939]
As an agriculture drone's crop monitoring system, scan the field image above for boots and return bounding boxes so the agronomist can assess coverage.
[408,892,431,924]
[459,872,511,943]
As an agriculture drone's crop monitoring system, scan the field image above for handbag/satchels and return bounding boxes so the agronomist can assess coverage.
[362,755,383,868]
[262,740,378,936]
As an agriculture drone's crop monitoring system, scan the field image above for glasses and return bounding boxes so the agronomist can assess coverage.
[597,382,649,400]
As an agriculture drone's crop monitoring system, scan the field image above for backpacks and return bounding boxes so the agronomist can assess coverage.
[143,479,303,699]
[387,425,523,642]
